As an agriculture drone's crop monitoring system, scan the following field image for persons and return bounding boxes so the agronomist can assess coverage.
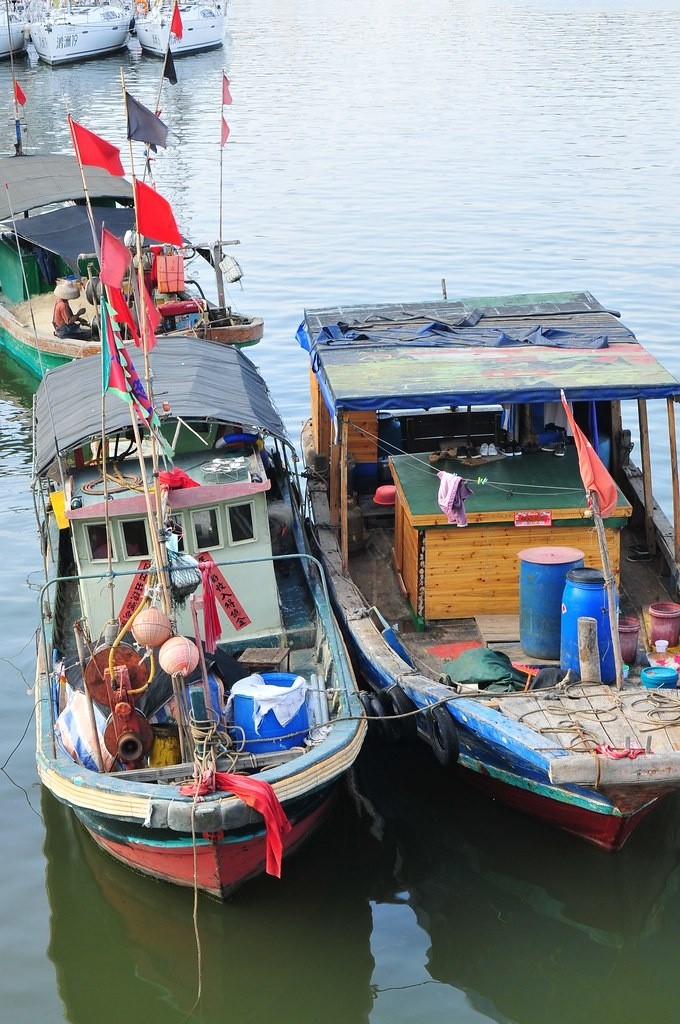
[52,281,92,340]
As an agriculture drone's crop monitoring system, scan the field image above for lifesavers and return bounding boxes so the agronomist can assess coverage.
[214,433,264,452]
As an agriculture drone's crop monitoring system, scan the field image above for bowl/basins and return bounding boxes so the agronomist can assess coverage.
[373,486,396,504]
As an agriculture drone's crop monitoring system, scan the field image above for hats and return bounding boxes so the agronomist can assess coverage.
[53,281,80,299]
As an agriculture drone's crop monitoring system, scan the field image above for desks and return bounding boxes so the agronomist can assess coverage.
[238,648,291,674]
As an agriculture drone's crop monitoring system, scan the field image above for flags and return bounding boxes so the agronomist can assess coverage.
[134,178,183,248]
[126,91,168,154]
[171,3,184,39]
[222,73,232,105]
[222,117,230,146]
[561,394,617,518]
[68,114,125,176]
[14,81,27,106]
[164,47,177,86]
[100,228,161,353]
[101,296,160,427]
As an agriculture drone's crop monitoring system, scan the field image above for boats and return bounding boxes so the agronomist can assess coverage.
[135,4,224,60]
[298,288,680,855]
[34,338,371,905]
[348,699,680,1023]
[0,6,29,60]
[43,794,376,1023]
[2,147,262,378]
[27,2,134,65]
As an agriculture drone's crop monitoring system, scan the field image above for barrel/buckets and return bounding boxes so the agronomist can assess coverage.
[641,666,679,689]
[623,665,629,678]
[649,602,680,648]
[618,617,640,665]
[231,672,309,753]
[148,724,185,768]
[378,457,391,481]
[655,640,668,652]
[377,412,401,456]
[520,559,584,660]
[561,567,619,685]
